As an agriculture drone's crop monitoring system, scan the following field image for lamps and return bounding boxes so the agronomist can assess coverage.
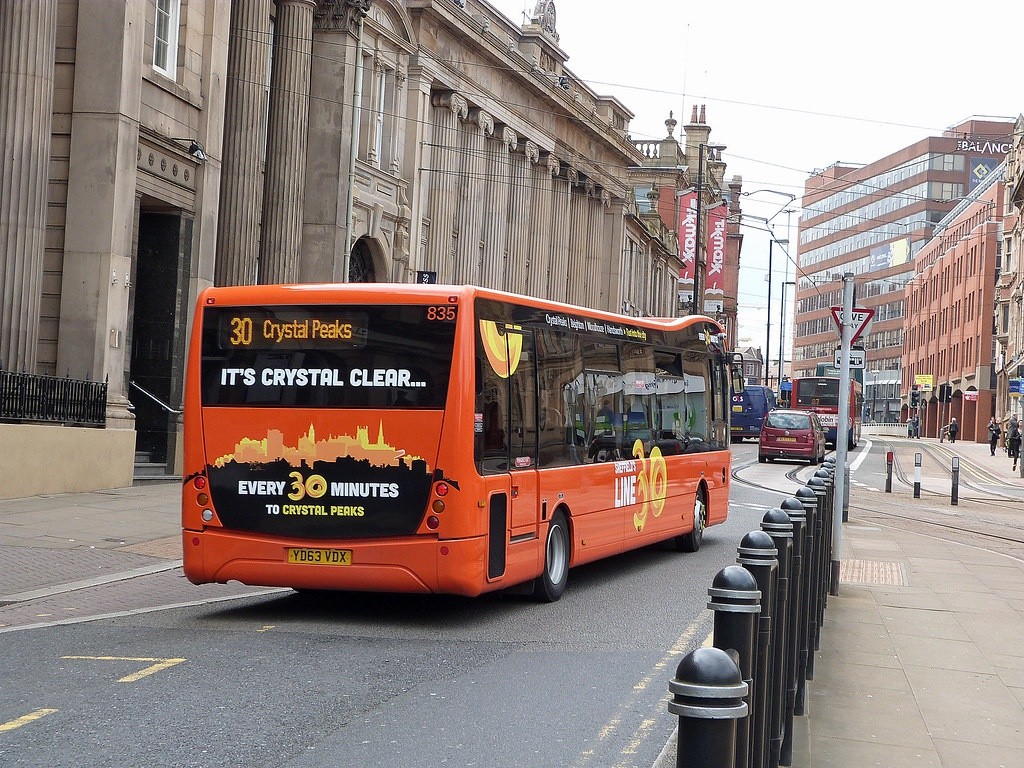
[170,136,205,160]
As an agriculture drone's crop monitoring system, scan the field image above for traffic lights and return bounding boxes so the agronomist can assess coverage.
[910,391,918,409]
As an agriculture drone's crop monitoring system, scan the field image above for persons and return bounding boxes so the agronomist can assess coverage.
[598,400,616,425]
[906,417,913,438]
[986,416,1001,456]
[913,417,920,439]
[1006,418,1023,471]
[949,418,959,443]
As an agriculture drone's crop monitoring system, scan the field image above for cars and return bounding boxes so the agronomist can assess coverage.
[756,407,829,465]
[586,427,726,463]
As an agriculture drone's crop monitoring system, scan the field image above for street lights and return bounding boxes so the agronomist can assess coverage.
[777,281,796,395]
[763,239,790,384]
[692,143,728,314]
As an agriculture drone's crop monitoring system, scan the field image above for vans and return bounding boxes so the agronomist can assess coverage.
[730,384,777,444]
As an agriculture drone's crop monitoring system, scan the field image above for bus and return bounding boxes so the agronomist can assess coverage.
[791,375,866,452]
[179,281,747,604]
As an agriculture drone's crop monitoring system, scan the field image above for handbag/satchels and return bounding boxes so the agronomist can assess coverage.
[948,434,951,441]
